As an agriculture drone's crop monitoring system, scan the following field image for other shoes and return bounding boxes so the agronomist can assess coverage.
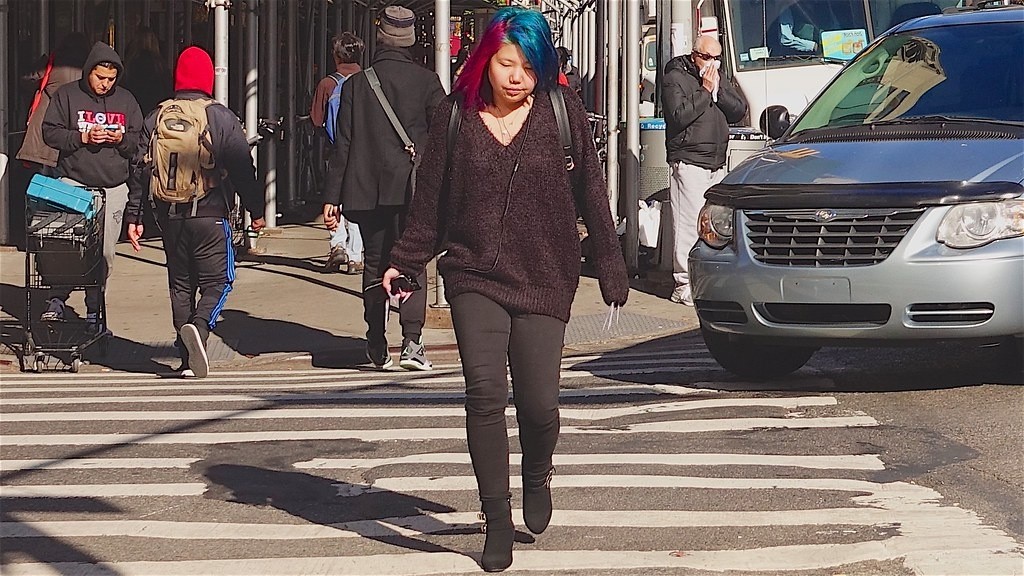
[321,250,345,273]
[175,321,210,379]
[346,260,364,275]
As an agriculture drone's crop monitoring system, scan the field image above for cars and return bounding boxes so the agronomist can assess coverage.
[688,6,1022,385]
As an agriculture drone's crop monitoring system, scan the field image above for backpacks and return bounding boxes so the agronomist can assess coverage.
[323,71,351,146]
[148,98,224,218]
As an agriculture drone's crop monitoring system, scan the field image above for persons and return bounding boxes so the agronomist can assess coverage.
[555,47,582,93]
[310,32,366,274]
[383,5,630,572]
[125,47,266,378]
[17,33,92,208]
[41,42,144,339]
[776,0,822,55]
[321,6,447,372]
[661,35,746,308]
[117,27,170,116]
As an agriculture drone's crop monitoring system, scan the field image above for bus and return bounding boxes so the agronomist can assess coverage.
[641,0,1008,141]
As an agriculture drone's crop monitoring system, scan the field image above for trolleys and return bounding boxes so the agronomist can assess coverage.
[23,187,110,372]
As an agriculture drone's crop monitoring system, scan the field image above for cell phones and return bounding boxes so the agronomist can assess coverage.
[390,275,413,295]
[329,205,334,217]
[104,126,117,132]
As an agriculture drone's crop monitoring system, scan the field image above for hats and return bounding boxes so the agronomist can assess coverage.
[377,5,415,47]
[174,47,215,97]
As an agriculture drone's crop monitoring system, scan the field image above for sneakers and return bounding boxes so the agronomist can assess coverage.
[671,282,694,306]
[40,298,69,324]
[353,341,394,368]
[399,337,433,371]
[83,312,100,334]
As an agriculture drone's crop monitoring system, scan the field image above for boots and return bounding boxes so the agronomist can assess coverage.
[522,465,555,534]
[477,501,516,571]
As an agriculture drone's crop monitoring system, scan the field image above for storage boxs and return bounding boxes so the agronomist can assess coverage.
[26,173,95,216]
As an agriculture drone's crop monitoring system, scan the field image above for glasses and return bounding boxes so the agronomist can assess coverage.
[692,49,722,61]
